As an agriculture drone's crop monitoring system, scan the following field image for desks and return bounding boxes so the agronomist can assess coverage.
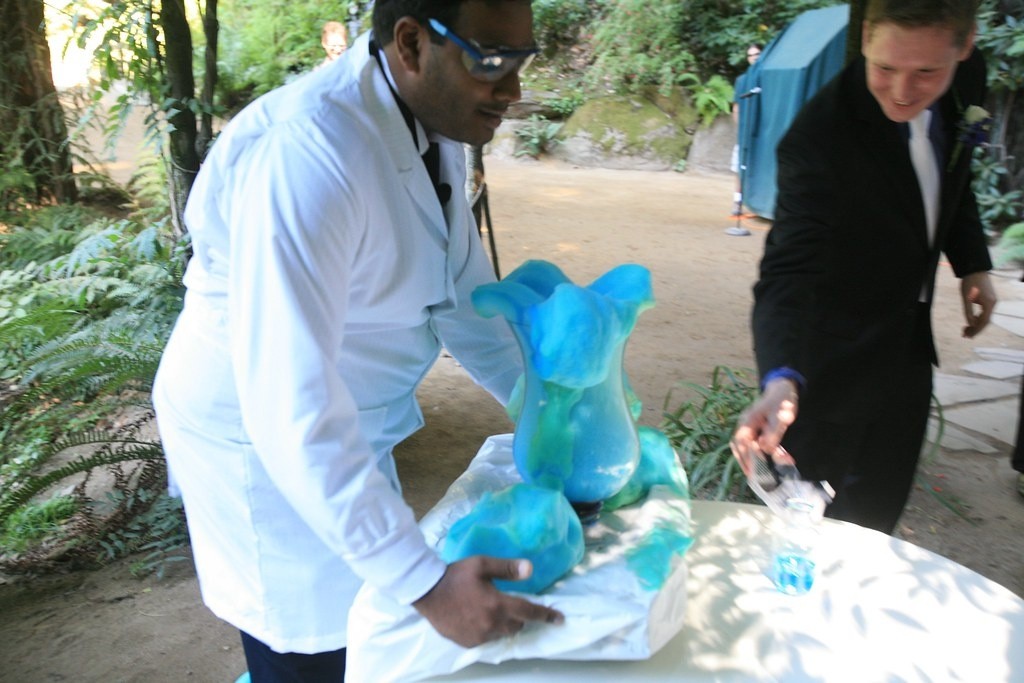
[418,498,1024,683]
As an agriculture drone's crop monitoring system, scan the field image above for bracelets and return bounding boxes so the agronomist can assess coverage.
[761,368,807,390]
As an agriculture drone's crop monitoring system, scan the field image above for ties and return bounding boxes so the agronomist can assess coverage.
[907,110,943,303]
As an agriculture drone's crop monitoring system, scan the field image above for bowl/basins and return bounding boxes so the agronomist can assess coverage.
[749,442,801,512]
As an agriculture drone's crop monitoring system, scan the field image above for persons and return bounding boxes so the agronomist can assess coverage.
[732,43,761,216]
[320,22,347,64]
[153,0,564,683]
[732,0,996,534]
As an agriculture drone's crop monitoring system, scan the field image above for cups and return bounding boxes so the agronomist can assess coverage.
[770,492,824,595]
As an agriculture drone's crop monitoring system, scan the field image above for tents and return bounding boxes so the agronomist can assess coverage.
[743,4,851,223]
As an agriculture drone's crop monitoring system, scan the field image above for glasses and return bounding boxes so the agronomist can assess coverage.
[415,13,539,82]
